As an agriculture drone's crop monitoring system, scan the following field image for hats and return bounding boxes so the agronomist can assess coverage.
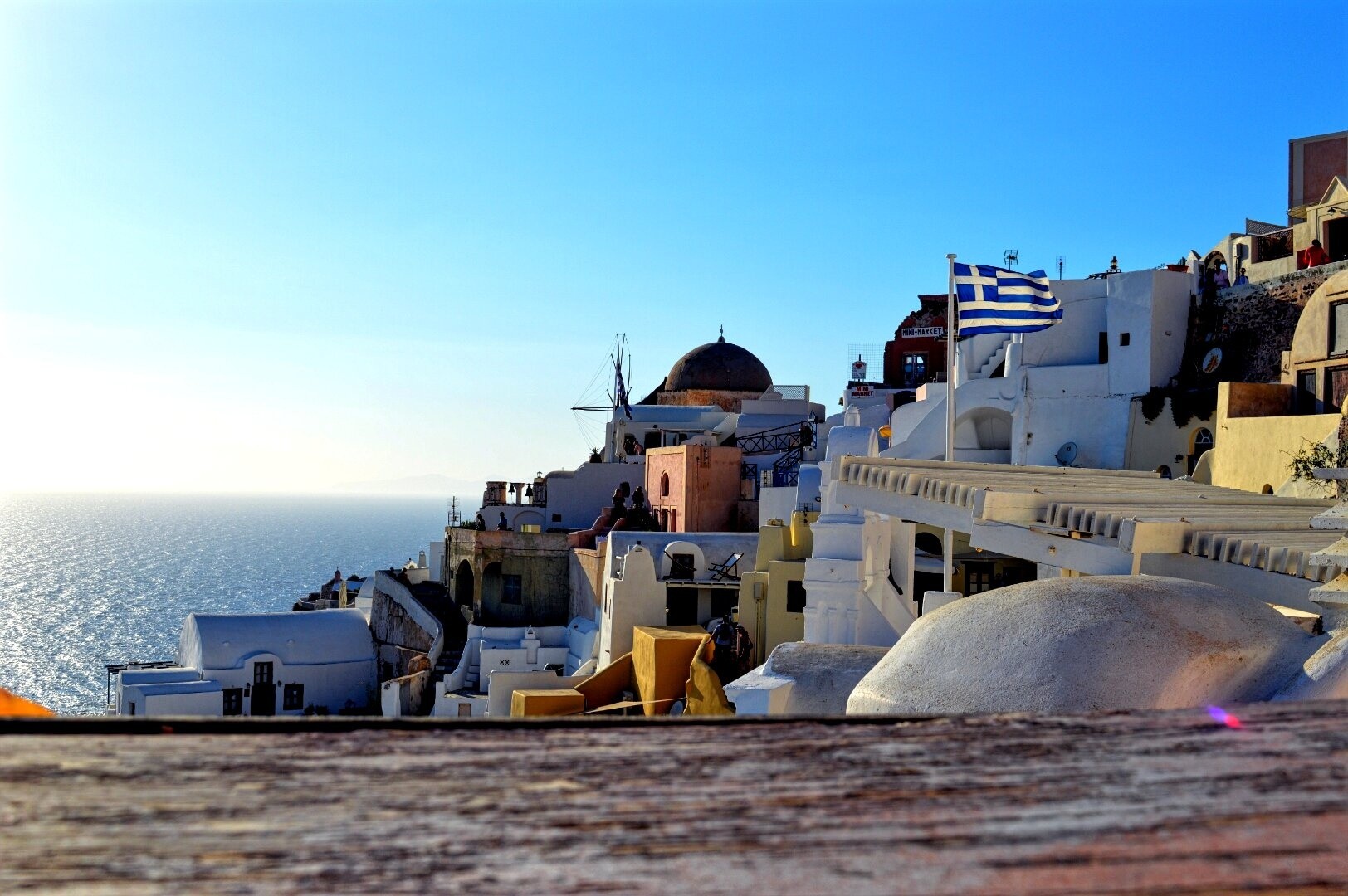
[735,624,744,629]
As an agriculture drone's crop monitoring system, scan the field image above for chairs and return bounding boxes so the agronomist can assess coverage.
[663,550,696,580]
[708,551,744,581]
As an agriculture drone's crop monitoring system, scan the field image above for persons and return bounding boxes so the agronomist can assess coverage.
[1232,268,1249,286]
[1305,239,1330,267]
[1213,264,1230,289]
[473,513,486,531]
[624,438,643,455]
[612,487,625,509]
[497,512,508,530]
[705,614,753,673]
[389,567,407,584]
[632,486,644,508]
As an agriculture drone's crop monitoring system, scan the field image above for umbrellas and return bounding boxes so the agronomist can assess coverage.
[339,580,347,608]
[615,418,626,464]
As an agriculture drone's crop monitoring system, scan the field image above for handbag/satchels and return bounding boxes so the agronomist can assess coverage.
[747,637,753,650]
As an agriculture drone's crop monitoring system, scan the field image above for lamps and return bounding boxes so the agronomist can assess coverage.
[1111,257,1118,269]
[1327,206,1338,215]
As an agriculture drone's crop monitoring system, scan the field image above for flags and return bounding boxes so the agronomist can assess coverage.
[953,262,1063,338]
